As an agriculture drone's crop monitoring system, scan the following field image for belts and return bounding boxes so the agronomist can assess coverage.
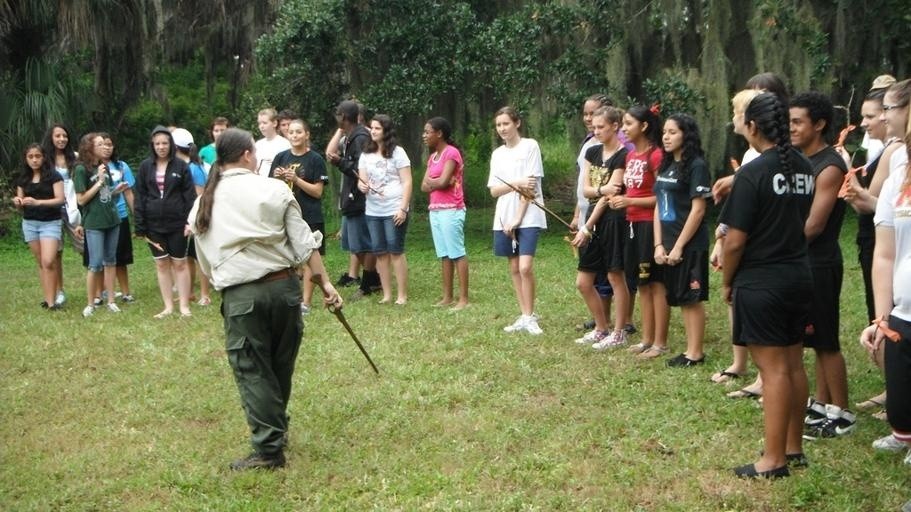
[264,269,289,282]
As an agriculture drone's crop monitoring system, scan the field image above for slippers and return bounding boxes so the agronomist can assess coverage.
[727,389,761,399]
[623,323,636,335]
[710,369,738,382]
[872,409,887,421]
[180,311,192,318]
[575,319,595,330]
[856,399,882,412]
[154,311,172,319]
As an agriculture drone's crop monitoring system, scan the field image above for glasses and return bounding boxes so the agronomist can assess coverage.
[334,110,342,116]
[881,105,903,111]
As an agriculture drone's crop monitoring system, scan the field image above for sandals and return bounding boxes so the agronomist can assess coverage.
[626,342,652,355]
[637,344,670,359]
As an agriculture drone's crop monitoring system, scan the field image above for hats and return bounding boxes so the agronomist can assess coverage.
[171,128,195,148]
[869,75,897,91]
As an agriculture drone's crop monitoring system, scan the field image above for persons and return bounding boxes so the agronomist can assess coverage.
[489,106,549,334]
[565,70,910,477]
[13,68,414,321]
[421,116,470,314]
[187,126,345,470]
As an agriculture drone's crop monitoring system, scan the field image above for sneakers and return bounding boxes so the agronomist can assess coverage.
[334,274,361,288]
[667,353,705,368]
[802,403,858,441]
[450,303,471,311]
[121,294,136,303]
[102,289,122,298]
[379,298,392,304]
[369,284,383,293]
[301,303,312,316]
[503,316,524,331]
[282,433,290,448]
[576,329,610,343]
[350,287,370,301]
[761,452,809,469]
[802,397,828,430]
[55,292,66,306]
[40,301,48,309]
[728,463,790,480]
[107,303,121,312]
[230,453,285,471]
[520,313,543,335]
[431,301,454,307]
[871,435,910,452]
[94,297,104,308]
[49,305,60,312]
[199,297,211,306]
[395,298,408,304]
[82,305,95,316]
[592,331,625,349]
[173,294,196,302]
